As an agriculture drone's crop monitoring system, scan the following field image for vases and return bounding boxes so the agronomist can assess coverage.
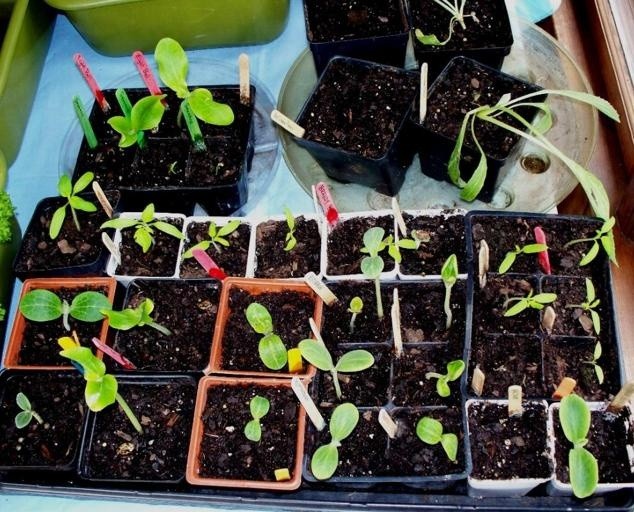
[287,54,427,194]
[38,1,292,58]
[302,2,411,79]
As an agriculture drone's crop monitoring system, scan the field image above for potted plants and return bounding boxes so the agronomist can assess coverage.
[66,35,256,214]
[0,185,634,497]
[410,56,620,221]
[408,1,514,74]
